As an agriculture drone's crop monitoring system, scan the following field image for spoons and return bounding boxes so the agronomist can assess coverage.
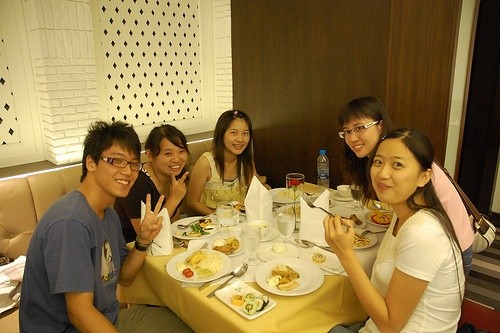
[207,263,248,299]
[177,220,200,229]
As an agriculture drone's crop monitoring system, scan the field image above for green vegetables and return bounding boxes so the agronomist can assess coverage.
[182,223,210,236]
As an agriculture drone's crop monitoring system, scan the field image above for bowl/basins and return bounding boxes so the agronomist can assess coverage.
[337,185,351,198]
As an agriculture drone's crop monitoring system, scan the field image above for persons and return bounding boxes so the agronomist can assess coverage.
[323,128,466,333]
[186,110,255,216]
[113,124,191,243]
[18,120,194,333]
[337,96,475,290]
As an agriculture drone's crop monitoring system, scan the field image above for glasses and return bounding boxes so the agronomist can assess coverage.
[338,120,380,138]
[100,155,143,172]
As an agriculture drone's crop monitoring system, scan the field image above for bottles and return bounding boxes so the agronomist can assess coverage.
[316,149,330,188]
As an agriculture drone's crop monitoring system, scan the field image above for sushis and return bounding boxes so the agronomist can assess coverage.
[231,293,269,314]
[312,253,326,263]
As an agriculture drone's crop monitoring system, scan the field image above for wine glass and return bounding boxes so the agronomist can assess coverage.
[350,184,363,211]
[272,206,296,254]
[215,201,234,230]
[238,224,261,266]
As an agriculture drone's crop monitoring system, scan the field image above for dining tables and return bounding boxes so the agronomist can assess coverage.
[116,181,394,333]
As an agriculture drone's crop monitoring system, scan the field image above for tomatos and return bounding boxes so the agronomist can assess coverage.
[183,268,194,277]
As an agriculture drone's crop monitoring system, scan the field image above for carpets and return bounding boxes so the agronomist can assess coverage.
[463,225,500,310]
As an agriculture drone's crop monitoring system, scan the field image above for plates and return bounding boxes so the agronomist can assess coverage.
[166,187,393,319]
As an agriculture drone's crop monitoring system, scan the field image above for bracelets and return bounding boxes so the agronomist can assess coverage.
[134,236,153,251]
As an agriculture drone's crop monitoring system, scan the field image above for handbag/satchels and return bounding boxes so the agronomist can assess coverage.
[468,212,496,253]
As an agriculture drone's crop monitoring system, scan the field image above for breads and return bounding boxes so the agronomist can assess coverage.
[373,214,393,224]
[213,245,235,255]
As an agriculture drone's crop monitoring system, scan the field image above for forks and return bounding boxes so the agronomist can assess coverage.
[361,229,386,236]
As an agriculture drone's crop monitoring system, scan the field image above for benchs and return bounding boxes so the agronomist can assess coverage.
[0,137,255,333]
